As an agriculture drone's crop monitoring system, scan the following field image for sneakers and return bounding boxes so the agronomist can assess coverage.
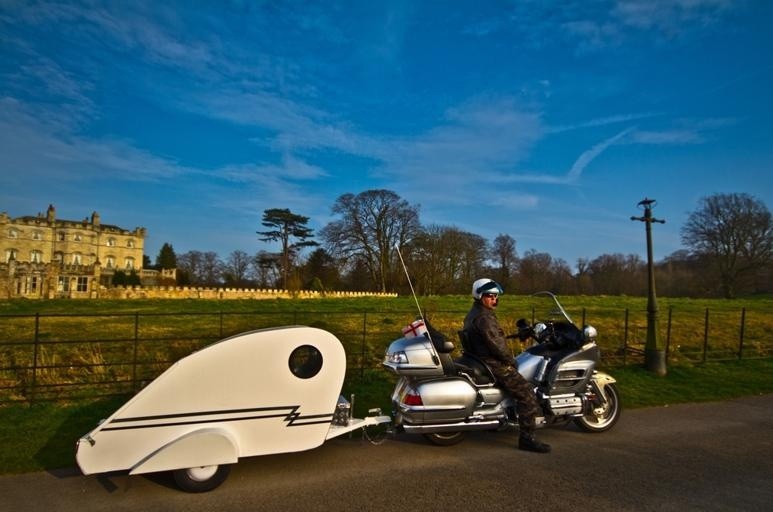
[518,434,551,453]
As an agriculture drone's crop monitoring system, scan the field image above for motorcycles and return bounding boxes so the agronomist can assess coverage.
[382,291,621,446]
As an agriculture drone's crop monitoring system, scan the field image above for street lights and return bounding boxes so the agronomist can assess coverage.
[637,198,666,376]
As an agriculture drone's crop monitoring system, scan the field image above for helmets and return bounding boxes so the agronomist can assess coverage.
[471,278,503,300]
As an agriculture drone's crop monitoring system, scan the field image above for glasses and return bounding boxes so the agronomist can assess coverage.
[482,293,498,298]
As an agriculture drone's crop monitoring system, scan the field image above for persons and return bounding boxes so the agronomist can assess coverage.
[462,277,553,454]
